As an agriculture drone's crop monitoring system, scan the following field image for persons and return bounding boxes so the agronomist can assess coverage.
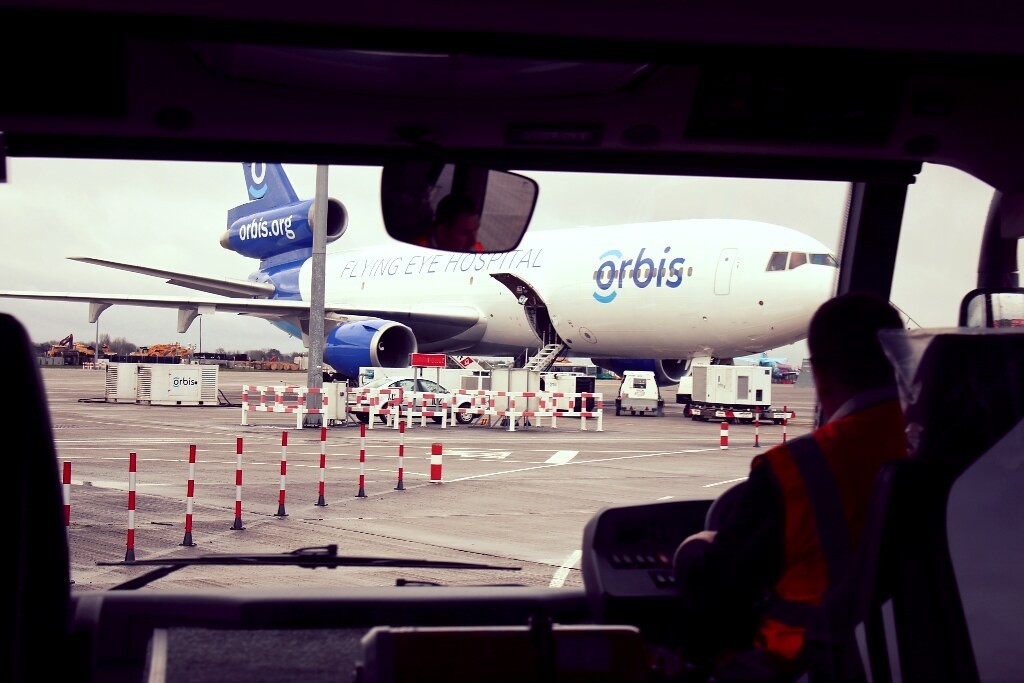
[409,194,484,252]
[673,294,924,683]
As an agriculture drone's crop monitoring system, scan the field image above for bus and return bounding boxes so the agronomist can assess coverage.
[45,334,97,366]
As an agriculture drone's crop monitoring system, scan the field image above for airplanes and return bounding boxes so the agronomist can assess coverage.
[1,157,856,390]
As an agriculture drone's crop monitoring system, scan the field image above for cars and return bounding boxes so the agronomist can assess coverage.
[348,376,485,426]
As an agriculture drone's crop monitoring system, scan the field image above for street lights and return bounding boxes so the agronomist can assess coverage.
[197,313,203,356]
